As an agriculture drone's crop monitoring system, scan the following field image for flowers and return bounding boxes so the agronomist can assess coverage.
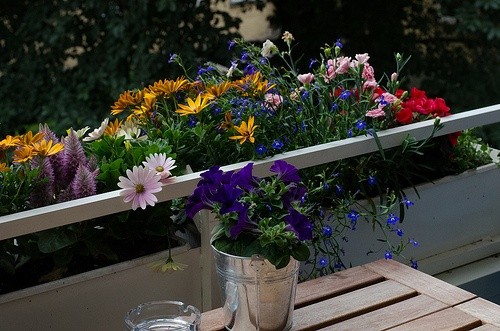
[184,159,314,270]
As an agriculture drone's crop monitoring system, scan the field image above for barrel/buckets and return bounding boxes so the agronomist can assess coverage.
[212,240,299,331]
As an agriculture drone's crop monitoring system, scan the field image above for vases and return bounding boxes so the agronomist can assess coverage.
[210,232,301,331]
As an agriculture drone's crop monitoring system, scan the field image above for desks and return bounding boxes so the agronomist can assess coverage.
[201,258,500,331]
